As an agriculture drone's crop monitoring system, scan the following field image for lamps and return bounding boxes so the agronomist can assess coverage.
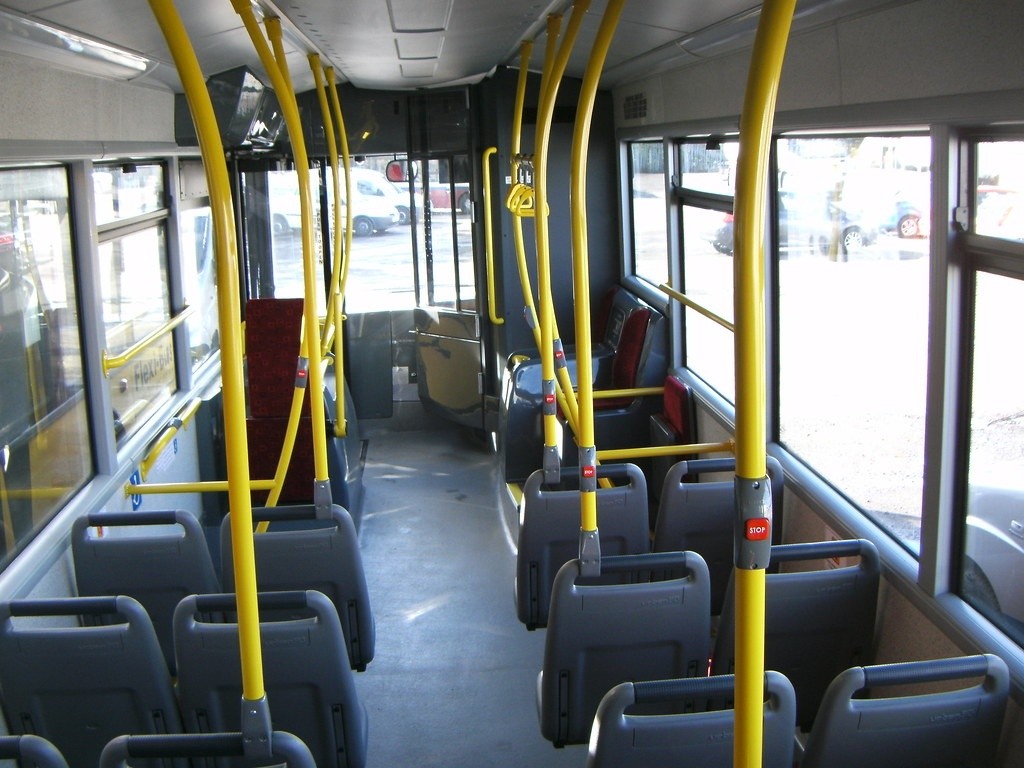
[599,0,917,93]
[0,5,209,95]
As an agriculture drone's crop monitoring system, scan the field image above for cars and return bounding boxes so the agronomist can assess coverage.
[714,156,922,258]
[860,447,1023,625]
[268,167,433,238]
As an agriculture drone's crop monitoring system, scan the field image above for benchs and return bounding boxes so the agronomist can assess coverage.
[516,457,1012,768]
[1,503,376,768]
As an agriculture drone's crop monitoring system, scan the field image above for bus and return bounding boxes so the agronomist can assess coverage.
[340,152,471,214]
[0,165,214,573]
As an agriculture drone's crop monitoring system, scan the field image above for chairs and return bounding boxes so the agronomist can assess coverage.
[554,305,668,450]
[244,298,312,418]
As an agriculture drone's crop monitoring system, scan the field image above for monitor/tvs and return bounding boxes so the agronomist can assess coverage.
[174,64,285,151]
